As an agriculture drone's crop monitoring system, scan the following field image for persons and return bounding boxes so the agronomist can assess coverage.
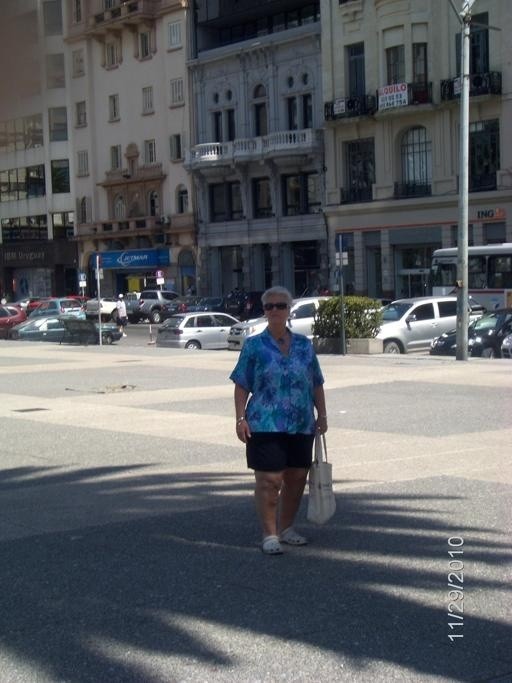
[228,290,233,298]
[229,284,328,553]
[233,286,239,297]
[238,287,249,319]
[118,294,130,337]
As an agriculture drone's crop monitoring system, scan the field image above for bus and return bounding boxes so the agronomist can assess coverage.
[421,241,512,309]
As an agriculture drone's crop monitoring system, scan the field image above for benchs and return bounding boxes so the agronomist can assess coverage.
[56,316,99,345]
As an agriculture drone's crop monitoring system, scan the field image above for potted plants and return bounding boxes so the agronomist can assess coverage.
[311,294,384,355]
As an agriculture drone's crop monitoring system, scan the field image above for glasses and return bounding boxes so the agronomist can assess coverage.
[262,302,289,312]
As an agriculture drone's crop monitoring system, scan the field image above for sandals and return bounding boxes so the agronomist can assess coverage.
[261,535,284,556]
[278,527,307,547]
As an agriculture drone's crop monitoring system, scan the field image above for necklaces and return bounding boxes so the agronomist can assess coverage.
[272,330,289,344]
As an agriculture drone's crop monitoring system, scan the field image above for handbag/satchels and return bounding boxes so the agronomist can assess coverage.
[307,431,337,527]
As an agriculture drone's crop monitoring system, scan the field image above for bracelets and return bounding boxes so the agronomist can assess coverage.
[235,417,245,424]
[318,414,327,419]
